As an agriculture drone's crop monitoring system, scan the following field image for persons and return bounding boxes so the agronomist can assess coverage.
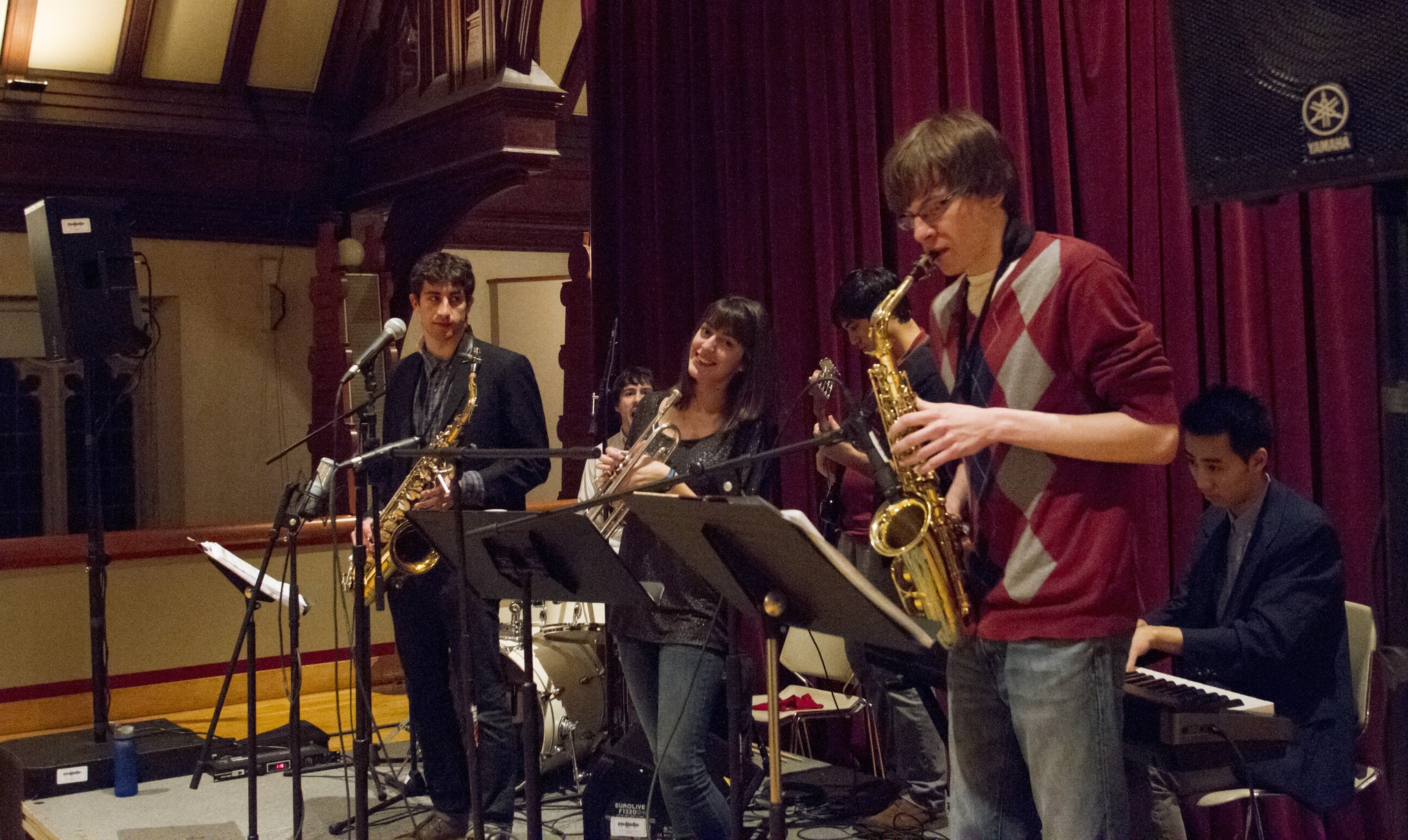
[812,263,961,837]
[1123,384,1366,839]
[880,108,1186,838]
[346,252,552,838]
[578,365,665,554]
[608,293,782,839]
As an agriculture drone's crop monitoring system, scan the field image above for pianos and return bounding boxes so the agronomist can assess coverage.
[1122,662,1295,772]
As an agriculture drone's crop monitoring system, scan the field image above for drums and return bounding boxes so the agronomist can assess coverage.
[483,625,615,802]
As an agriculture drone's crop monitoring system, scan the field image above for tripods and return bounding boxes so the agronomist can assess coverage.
[328,694,433,836]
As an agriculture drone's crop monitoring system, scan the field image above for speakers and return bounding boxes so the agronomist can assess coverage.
[1165,0,1408,203]
[22,194,154,363]
[339,271,388,449]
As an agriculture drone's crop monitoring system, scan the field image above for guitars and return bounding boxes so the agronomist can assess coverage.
[806,357,851,552]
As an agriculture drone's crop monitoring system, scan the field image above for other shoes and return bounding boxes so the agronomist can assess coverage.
[391,809,468,840]
[855,796,949,834]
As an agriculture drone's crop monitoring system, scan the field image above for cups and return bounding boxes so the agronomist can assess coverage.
[115,723,139,797]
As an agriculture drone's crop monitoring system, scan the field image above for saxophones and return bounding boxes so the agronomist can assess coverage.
[865,248,983,654]
[337,346,483,610]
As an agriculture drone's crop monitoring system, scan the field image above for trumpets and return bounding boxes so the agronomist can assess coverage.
[571,389,686,542]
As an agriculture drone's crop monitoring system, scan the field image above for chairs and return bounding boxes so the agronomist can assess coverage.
[1180,600,1382,839]
[748,625,886,781]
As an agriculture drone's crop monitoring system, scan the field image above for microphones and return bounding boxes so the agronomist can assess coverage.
[340,318,408,384]
[298,456,338,516]
[838,381,898,499]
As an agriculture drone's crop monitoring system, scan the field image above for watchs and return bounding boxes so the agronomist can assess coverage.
[659,467,676,494]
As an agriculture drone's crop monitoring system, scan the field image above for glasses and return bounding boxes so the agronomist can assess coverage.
[895,183,968,232]
